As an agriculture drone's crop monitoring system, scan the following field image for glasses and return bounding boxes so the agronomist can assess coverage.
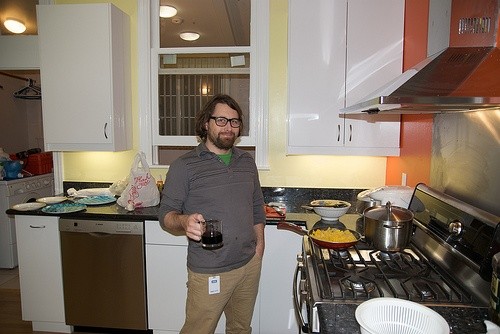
[209,117,242,128]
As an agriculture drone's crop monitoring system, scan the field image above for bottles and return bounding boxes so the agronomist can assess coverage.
[489,253,500,313]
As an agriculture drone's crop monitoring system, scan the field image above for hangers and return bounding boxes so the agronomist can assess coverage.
[13,77,41,99]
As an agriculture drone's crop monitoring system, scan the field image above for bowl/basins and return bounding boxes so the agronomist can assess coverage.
[313,218,346,228]
[354,297,450,334]
[310,199,351,222]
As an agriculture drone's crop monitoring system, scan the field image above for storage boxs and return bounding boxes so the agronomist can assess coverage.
[13,153,52,175]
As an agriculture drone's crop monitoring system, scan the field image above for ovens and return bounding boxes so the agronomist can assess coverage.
[293,261,320,334]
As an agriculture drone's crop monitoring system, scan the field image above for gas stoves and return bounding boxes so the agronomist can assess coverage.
[302,182,500,310]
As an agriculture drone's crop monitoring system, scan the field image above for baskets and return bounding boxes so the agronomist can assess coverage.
[355,297,450,334]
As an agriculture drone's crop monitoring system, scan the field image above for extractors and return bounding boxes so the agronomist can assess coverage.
[339,0,500,116]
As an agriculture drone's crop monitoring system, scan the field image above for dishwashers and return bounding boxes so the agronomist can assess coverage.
[58,217,151,334]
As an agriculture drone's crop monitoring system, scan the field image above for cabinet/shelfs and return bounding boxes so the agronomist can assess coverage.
[144,218,307,334]
[283,0,405,157]
[35,3,134,152]
[14,214,74,334]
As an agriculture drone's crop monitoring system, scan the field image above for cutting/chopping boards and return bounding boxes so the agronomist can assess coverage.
[264,205,286,218]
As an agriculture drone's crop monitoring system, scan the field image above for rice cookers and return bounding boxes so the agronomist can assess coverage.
[356,185,414,217]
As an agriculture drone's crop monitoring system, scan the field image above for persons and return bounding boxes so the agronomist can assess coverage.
[159,93,266,334]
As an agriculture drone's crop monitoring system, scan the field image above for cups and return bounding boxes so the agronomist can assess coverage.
[198,218,224,247]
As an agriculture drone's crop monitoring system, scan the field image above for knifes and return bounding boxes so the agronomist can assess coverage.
[273,207,285,216]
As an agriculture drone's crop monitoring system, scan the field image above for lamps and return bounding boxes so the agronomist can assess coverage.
[160,6,177,18]
[3,19,26,33]
[179,32,200,41]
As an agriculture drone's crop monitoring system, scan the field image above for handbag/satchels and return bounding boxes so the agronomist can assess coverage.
[117,152,160,207]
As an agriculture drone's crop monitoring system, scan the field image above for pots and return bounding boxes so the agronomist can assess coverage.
[277,221,362,249]
[362,203,414,252]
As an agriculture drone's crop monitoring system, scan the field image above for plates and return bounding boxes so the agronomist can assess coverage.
[11,185,117,214]
